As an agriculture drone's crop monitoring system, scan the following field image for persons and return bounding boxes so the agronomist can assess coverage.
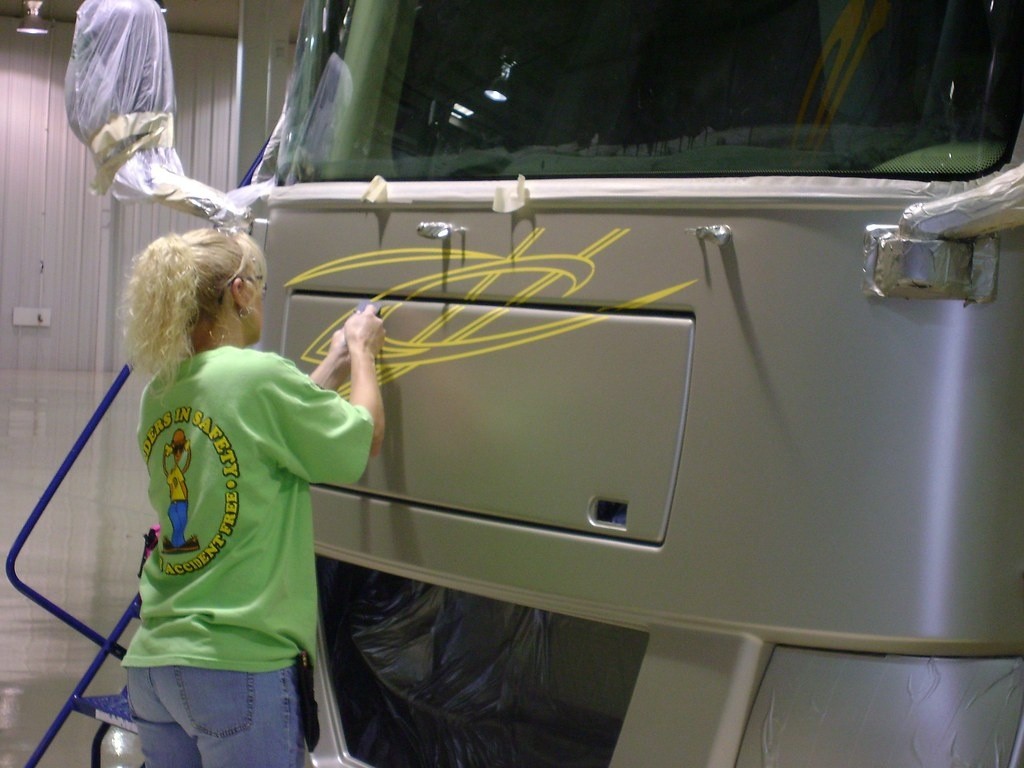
[118,226,386,768]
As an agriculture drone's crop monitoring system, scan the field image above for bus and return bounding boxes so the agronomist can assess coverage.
[223,0,1023,766]
